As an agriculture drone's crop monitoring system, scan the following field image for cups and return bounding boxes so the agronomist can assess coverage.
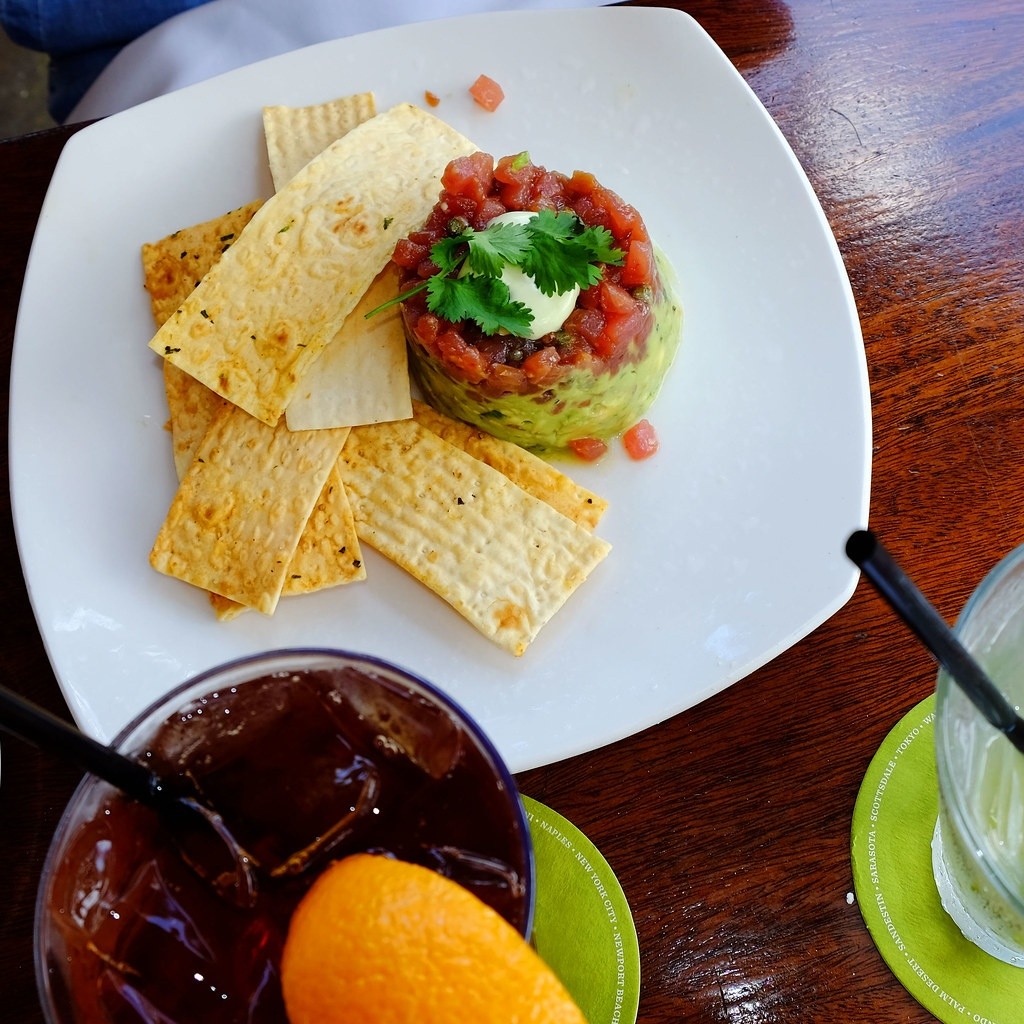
[933,544,1024,970]
[34,650,535,1024]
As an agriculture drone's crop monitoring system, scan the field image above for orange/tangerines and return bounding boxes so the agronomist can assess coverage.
[281,860,587,1024]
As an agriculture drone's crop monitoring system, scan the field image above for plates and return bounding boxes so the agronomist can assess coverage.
[12,4,874,779]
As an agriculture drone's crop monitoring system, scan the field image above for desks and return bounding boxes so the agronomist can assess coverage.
[0,0,1024,1024]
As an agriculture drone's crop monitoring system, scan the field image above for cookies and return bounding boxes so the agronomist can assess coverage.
[143,93,613,656]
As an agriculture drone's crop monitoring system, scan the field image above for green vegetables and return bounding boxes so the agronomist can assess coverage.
[364,211,625,336]
